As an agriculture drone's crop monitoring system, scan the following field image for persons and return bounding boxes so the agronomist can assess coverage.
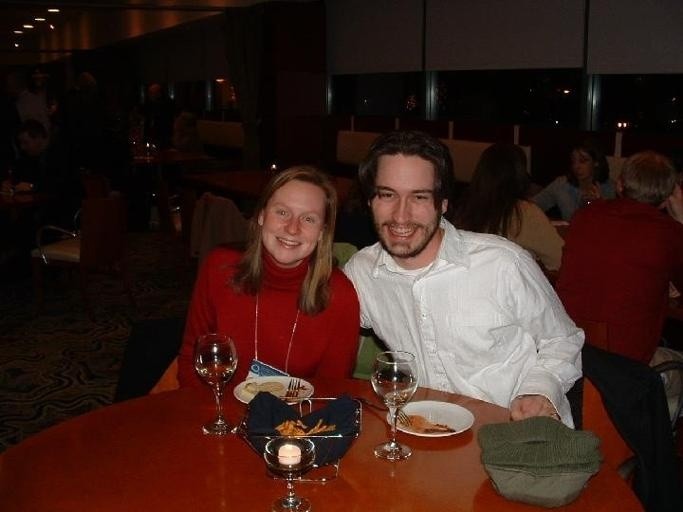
[527,137,619,221]
[553,151,683,426]
[175,164,361,389]
[15,62,54,131]
[1,119,87,281]
[447,141,565,272]
[341,130,587,431]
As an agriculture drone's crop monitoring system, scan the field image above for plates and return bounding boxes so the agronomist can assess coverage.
[385,398,477,440]
[233,376,315,406]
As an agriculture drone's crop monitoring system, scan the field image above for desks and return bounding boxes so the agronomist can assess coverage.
[206,170,355,208]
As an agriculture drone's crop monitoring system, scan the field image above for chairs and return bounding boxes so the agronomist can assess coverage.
[32,198,128,315]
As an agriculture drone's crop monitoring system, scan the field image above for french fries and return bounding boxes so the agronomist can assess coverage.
[275,418,337,437]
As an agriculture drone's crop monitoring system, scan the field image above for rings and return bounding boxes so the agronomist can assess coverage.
[552,412,561,421]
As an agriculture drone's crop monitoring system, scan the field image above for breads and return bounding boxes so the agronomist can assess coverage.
[247,382,285,393]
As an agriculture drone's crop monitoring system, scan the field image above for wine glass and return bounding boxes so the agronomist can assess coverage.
[191,332,239,436]
[261,438,315,512]
[372,348,417,460]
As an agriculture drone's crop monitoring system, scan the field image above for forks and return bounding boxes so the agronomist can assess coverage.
[281,378,305,402]
[360,395,416,429]
